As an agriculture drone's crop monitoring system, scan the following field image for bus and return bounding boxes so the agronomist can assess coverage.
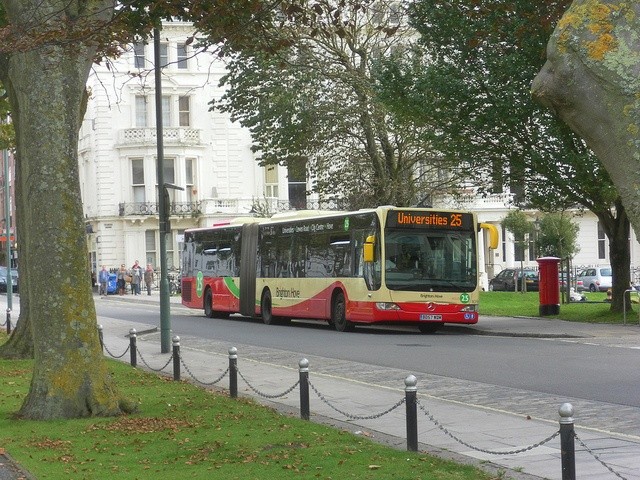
[182,205,499,332]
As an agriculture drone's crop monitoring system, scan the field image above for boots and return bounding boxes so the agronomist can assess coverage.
[122,289,124,295]
[119,288,123,296]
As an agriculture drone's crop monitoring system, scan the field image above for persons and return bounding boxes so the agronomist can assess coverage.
[116,264,129,296]
[98,265,110,296]
[143,264,155,296]
[131,260,143,294]
[130,266,141,296]
[374,254,399,273]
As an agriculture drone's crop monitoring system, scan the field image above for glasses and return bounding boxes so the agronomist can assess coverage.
[122,266,125,267]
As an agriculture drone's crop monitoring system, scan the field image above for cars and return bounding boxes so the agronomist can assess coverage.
[0,266,19,293]
[488,269,539,292]
[579,267,612,292]
[559,271,584,293]
